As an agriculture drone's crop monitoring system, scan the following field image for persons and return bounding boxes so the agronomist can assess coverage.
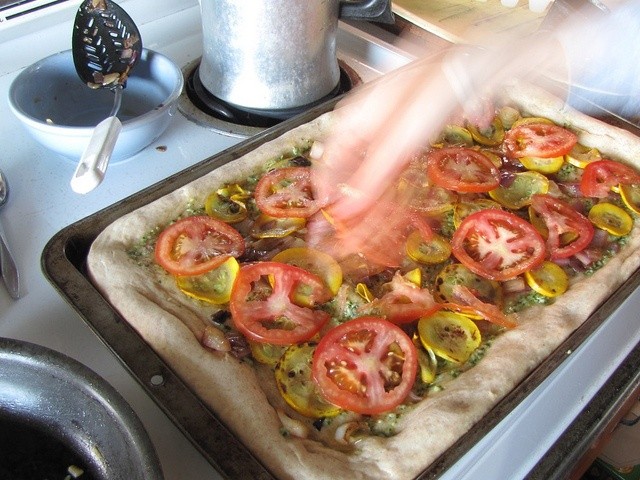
[308,0,640,280]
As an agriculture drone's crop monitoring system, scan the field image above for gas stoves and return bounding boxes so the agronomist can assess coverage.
[0,0,640,475]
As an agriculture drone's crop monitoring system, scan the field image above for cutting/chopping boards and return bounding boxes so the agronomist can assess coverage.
[386,0,543,50]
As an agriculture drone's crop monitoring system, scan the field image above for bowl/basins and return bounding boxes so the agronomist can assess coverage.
[7,44,185,165]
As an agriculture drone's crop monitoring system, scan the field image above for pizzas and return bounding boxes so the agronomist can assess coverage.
[84,77,639,479]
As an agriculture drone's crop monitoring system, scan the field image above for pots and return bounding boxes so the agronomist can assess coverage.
[0,334,167,479]
[199,0,396,110]
